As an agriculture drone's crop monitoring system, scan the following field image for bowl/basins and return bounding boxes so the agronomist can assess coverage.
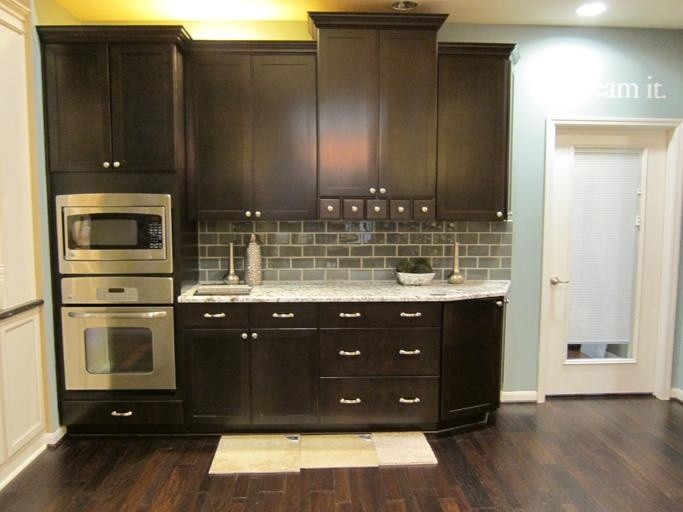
[394,267,437,285]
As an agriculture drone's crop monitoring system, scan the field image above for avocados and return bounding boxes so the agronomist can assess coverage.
[396,258,432,273]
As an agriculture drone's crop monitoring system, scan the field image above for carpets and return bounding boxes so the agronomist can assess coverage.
[208,432,439,475]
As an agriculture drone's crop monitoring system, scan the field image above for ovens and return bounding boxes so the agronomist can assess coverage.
[59,308,176,391]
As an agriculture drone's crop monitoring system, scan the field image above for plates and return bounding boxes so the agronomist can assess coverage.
[196,286,251,295]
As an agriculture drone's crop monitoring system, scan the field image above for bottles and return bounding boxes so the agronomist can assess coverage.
[448,240,464,284]
[220,242,241,283]
[243,234,263,285]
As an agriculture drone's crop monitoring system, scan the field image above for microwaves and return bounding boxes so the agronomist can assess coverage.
[51,192,171,274]
